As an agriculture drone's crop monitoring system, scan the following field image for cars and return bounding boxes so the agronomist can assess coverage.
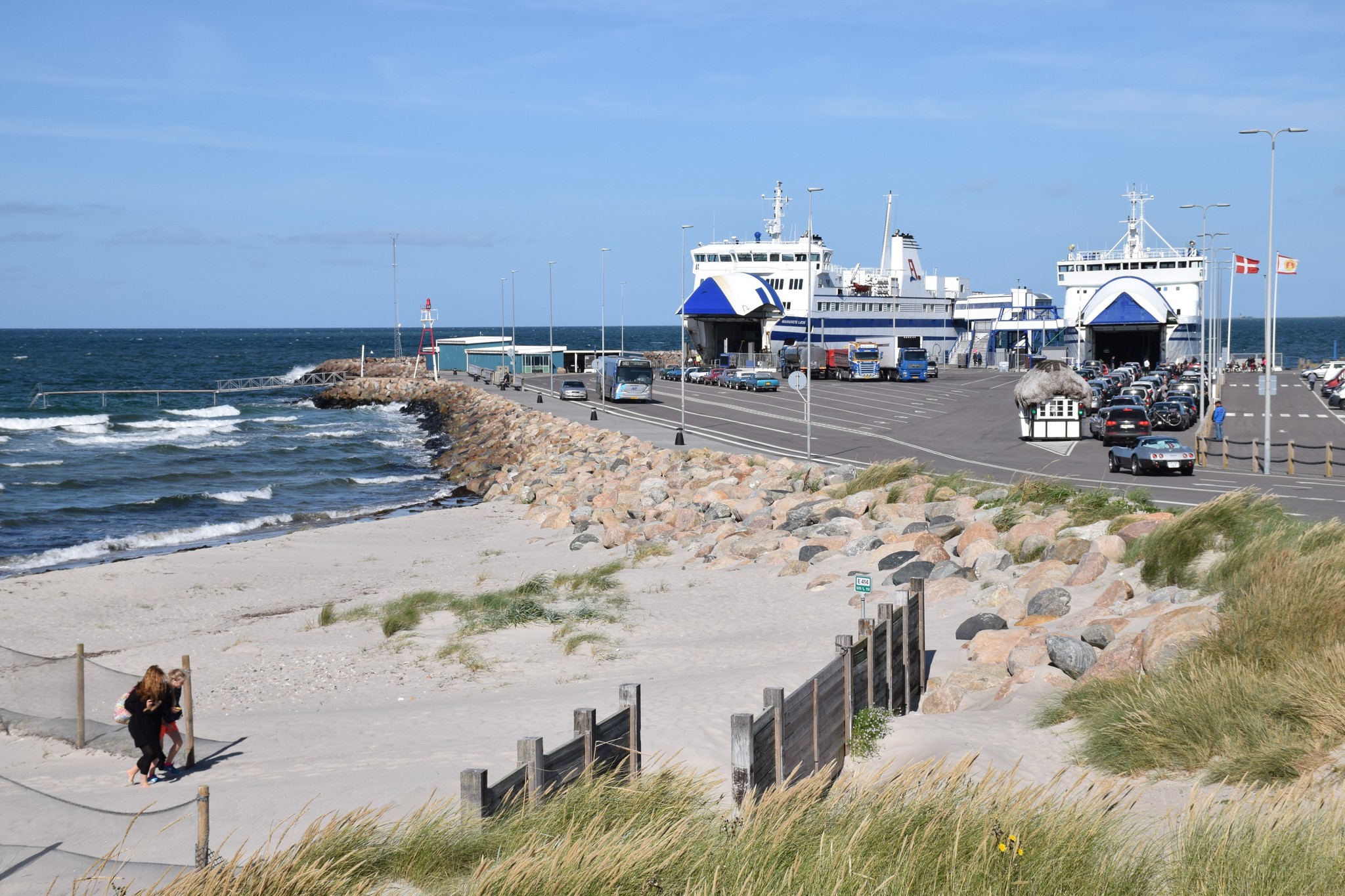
[660,363,780,393]
[1071,359,1210,430]
[1089,407,1111,440]
[1108,435,1196,476]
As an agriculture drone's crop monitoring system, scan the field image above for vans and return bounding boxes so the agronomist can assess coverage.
[1321,368,1345,410]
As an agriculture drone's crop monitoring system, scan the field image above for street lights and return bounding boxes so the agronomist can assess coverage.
[807,188,824,460]
[1240,127,1308,473]
[1198,233,1234,400]
[680,225,693,432]
[1181,203,1231,424]
[501,248,626,411]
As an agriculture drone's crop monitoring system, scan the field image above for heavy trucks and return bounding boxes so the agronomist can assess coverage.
[778,335,931,382]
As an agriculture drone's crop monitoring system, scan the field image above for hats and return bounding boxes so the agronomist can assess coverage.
[1213,401,1222,405]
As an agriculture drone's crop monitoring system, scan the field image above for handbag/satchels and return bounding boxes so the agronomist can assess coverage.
[113,688,135,725]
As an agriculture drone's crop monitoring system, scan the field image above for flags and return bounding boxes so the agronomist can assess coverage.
[1277,255,1299,274]
[1235,256,1260,274]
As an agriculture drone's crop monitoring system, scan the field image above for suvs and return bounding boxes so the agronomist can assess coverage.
[568,361,585,373]
[927,360,938,377]
[559,379,588,401]
[1301,360,1345,379]
[1103,404,1153,446]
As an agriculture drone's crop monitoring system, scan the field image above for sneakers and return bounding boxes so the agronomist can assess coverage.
[147,775,164,783]
[164,761,180,774]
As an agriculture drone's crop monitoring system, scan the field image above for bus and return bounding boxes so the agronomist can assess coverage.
[596,355,656,403]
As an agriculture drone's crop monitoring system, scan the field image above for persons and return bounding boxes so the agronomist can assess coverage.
[728,362,736,374]
[973,347,982,367]
[1159,356,1197,374]
[1144,358,1150,377]
[125,666,187,788]
[1212,401,1226,442]
[1308,371,1317,391]
[1111,356,1115,372]
[1229,354,1265,374]
[692,354,702,367]
[1119,361,1124,368]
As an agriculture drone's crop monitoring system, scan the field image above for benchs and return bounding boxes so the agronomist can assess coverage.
[464,364,524,391]
[532,369,543,373]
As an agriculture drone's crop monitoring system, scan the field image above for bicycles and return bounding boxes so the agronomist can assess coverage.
[1149,407,1181,428]
[1226,362,1274,374]
